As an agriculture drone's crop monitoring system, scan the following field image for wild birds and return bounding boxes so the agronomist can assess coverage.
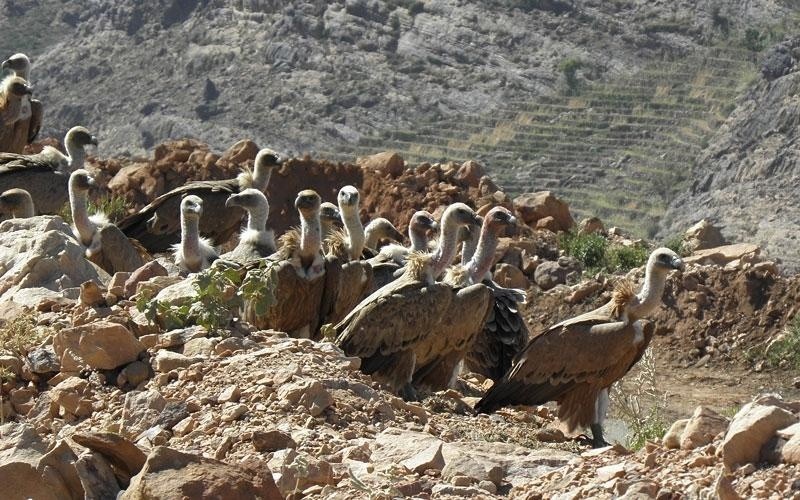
[0,48,685,452]
